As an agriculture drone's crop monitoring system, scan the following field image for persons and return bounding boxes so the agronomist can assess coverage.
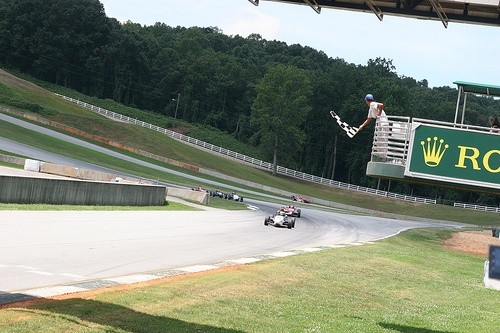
[278,208,285,217]
[288,202,295,209]
[216,188,220,192]
[489,114,500,133]
[358,94,389,157]
[197,186,202,190]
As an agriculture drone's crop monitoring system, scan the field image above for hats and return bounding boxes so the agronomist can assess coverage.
[365,94,374,100]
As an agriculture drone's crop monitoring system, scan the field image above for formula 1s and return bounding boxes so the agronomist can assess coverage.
[264,213,295,229]
[291,195,311,203]
[191,186,244,203]
[278,205,301,217]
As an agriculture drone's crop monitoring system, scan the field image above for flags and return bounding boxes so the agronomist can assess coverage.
[330,111,358,138]
